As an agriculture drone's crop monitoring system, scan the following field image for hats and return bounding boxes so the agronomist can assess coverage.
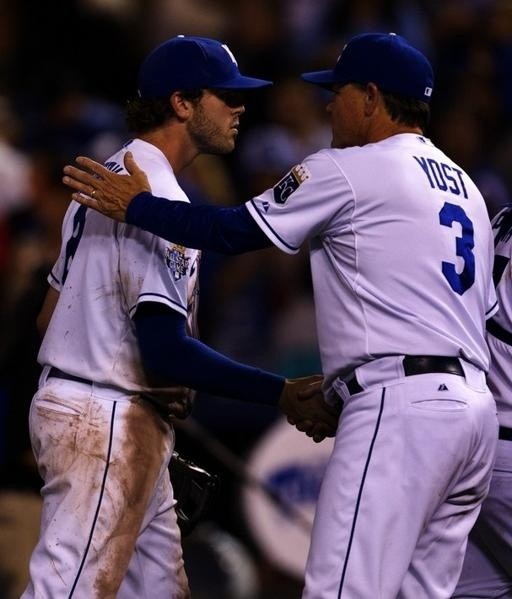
[301,32,434,103]
[139,35,273,100]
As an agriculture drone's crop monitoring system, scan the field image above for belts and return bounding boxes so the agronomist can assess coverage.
[48,368,93,385]
[329,356,466,414]
[499,427,512,440]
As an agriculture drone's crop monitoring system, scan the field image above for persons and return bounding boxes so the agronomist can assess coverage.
[62,32,497,599]
[19,33,339,599]
[471,206,512,599]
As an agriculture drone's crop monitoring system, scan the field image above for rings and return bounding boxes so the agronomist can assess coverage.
[92,191,96,198]
